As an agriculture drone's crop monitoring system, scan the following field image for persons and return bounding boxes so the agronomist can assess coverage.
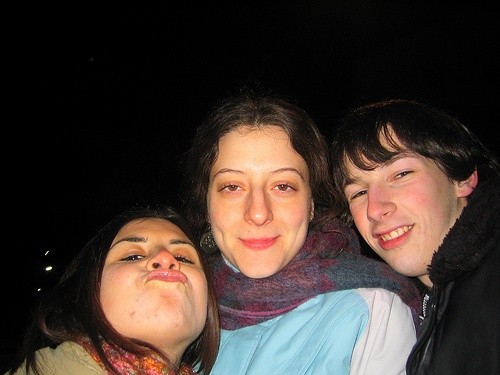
[332,97,500,375]
[175,96,423,375]
[1,202,221,375]
